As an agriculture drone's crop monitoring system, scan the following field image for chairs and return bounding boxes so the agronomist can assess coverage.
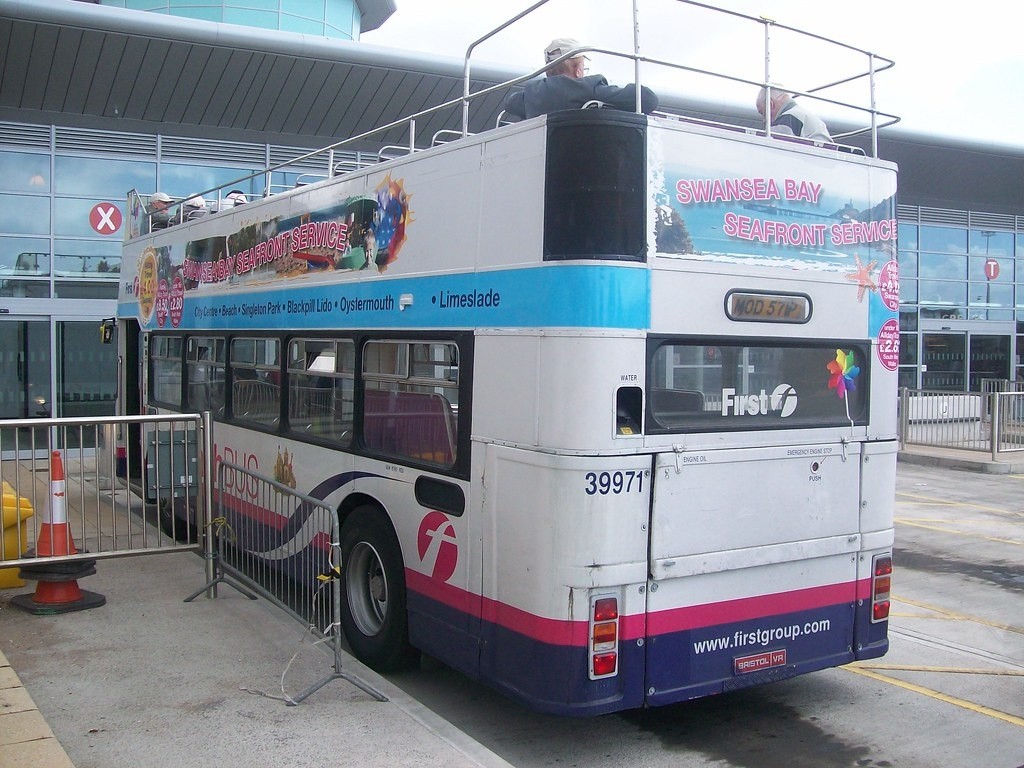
[149,99,622,234]
[649,389,706,413]
[152,370,457,467]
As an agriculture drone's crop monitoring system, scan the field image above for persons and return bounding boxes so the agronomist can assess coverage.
[234,361,280,415]
[505,38,658,120]
[140,189,247,236]
[756,82,835,143]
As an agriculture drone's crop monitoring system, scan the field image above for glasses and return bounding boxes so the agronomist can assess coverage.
[583,68,589,75]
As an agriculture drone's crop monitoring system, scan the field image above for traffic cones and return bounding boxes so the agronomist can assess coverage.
[7,450,108,617]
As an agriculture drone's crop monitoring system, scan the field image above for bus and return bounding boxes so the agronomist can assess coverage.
[100,0,898,715]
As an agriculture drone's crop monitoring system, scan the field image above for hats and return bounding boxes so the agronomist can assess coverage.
[185,194,205,207]
[148,193,175,203]
[544,38,591,61]
